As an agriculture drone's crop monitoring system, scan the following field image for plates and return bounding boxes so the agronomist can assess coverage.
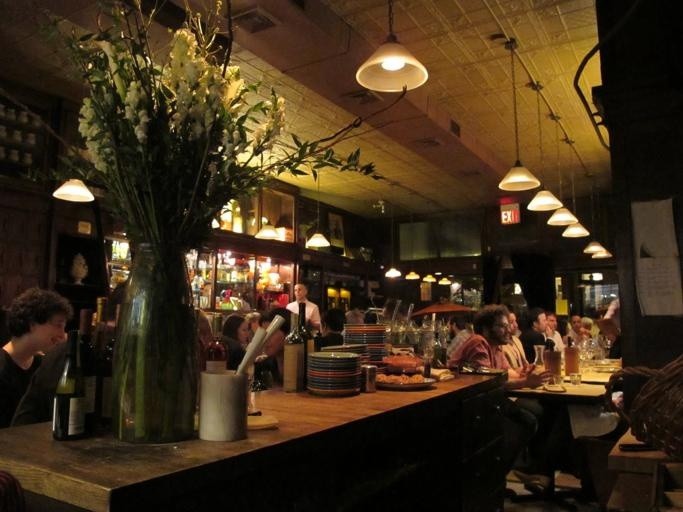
[306,351,362,398]
[372,377,436,390]
[321,343,369,367]
[343,322,388,375]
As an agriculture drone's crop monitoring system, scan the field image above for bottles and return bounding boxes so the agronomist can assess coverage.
[283,324,305,392]
[101,303,123,417]
[564,336,580,376]
[543,339,561,382]
[298,303,309,342]
[306,336,318,353]
[425,358,431,379]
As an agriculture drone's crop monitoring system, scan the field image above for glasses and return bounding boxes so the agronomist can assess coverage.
[497,322,511,329]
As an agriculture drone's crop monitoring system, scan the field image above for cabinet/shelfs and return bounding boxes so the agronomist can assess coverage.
[106,179,299,333]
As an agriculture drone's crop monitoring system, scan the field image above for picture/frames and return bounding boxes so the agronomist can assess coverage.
[327,213,345,248]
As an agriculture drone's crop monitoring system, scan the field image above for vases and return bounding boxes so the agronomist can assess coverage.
[118,247,197,446]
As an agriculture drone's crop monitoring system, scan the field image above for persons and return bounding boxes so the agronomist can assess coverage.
[602,296,622,357]
[498,311,543,466]
[10,280,131,425]
[344,303,383,328]
[516,308,552,365]
[284,284,322,334]
[445,317,470,364]
[566,315,591,348]
[260,309,302,387]
[222,314,247,344]
[544,314,564,359]
[314,309,347,352]
[0,289,71,427]
[449,305,537,491]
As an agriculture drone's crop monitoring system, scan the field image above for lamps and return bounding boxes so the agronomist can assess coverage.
[499,193,520,226]
[384,267,402,278]
[583,178,605,256]
[53,172,95,203]
[498,48,541,192]
[548,123,579,226]
[406,269,421,280]
[423,271,437,283]
[438,274,452,287]
[527,92,564,211]
[562,147,591,238]
[593,187,614,261]
[356,0,428,92]
[306,227,332,248]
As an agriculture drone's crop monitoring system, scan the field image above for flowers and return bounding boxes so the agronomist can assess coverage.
[16,0,428,437]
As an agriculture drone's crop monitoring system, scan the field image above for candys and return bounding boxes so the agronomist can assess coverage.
[77,309,98,429]
[96,297,109,416]
[52,331,88,441]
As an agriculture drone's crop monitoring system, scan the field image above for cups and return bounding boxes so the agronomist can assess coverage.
[569,373,582,388]
[197,371,248,441]
[553,374,565,385]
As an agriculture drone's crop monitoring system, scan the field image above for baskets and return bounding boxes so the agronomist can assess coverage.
[606,356,683,459]
